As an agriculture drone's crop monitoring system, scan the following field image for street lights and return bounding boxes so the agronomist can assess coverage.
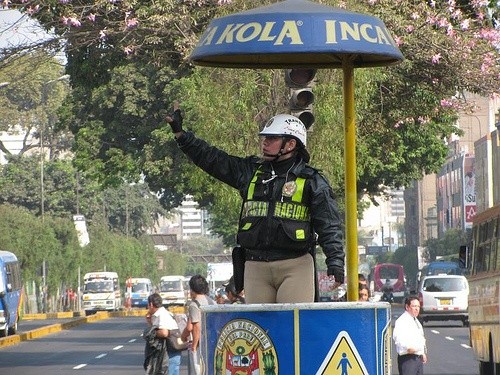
[39,74,70,287]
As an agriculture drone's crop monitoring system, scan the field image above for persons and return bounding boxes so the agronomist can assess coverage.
[379,279,395,301]
[180,275,214,375]
[393,297,427,375]
[145,293,181,375]
[166,99,346,303]
[214,275,245,304]
[337,274,368,302]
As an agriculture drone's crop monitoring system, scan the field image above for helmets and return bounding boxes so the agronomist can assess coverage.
[258,113,311,162]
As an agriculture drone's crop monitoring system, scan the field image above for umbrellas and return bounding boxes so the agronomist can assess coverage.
[190,0,404,69]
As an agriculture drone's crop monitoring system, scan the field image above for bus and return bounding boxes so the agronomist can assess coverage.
[370,263,406,300]
[126,278,152,308]
[0,250,22,337]
[159,276,186,305]
[468,205,500,375]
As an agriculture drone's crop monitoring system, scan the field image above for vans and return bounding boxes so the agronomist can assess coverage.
[81,272,121,316]
[416,261,469,324]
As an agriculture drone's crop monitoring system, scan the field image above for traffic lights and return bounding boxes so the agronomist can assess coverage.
[286,68,316,133]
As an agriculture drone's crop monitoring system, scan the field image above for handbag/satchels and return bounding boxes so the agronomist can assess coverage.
[167,329,189,352]
